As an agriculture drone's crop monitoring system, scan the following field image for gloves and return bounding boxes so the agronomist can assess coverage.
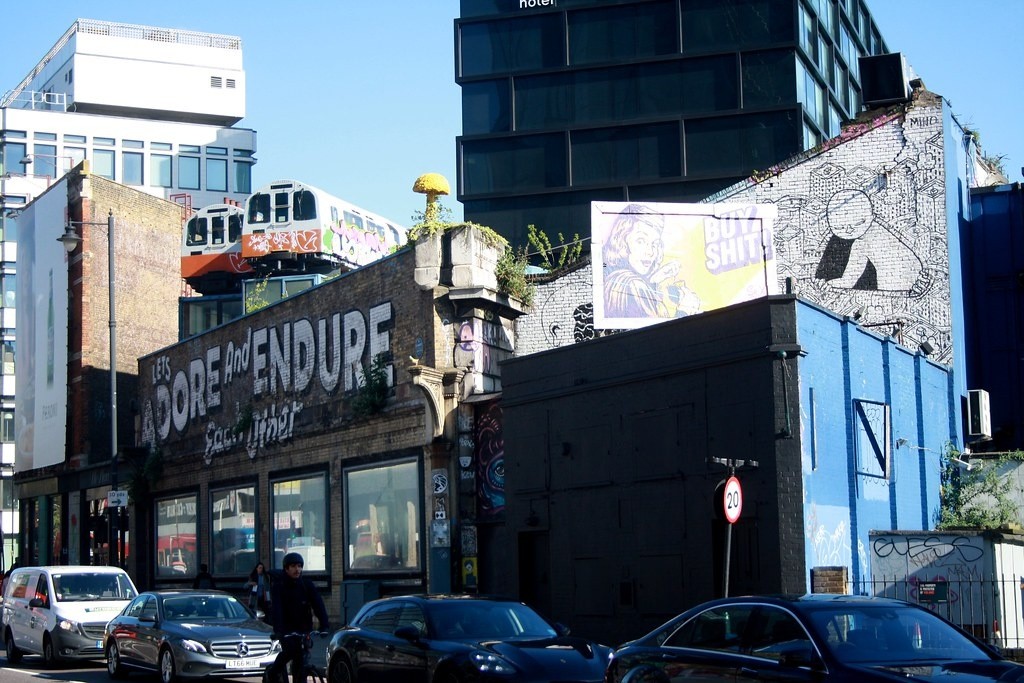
[316,627,329,639]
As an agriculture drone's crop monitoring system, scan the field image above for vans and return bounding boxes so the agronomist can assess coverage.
[2,565,144,669]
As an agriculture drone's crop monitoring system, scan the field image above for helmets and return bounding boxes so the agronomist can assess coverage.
[283,553,304,568]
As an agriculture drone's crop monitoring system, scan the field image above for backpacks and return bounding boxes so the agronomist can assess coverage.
[199,573,211,589]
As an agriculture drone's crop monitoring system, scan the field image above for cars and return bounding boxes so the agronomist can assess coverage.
[102,589,290,683]
[605,593,1022,683]
[323,595,614,682]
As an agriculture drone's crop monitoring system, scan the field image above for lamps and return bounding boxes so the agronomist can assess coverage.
[892,326,901,339]
[920,341,934,355]
[853,307,865,320]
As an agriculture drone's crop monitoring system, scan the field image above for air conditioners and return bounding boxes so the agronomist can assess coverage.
[967,389,992,437]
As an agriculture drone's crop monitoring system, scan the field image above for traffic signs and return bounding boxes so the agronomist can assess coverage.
[108,491,129,508]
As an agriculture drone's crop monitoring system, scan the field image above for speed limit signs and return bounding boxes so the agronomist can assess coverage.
[722,476,743,523]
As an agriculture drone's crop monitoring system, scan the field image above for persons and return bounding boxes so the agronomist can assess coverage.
[242,553,329,683]
[193,564,217,590]
[1,556,19,597]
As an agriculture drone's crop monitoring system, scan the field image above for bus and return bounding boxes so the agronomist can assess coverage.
[179,201,248,297]
[240,180,410,273]
